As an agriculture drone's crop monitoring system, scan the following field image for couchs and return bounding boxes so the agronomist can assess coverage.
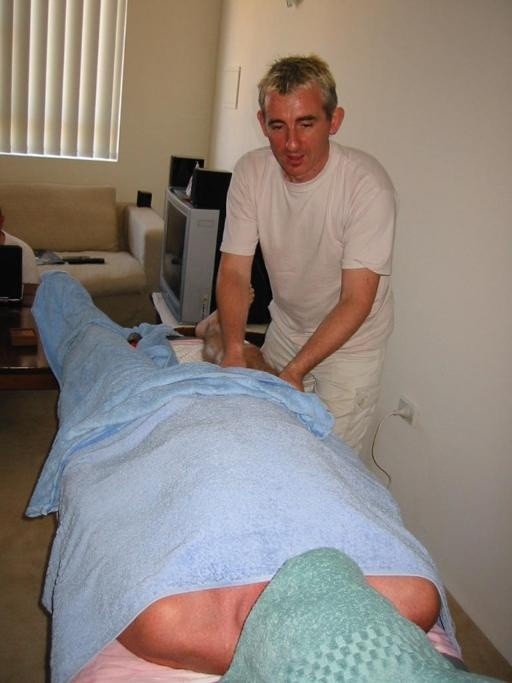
[1,182,165,370]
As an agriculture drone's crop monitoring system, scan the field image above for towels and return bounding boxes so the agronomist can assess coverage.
[218,550,500,683]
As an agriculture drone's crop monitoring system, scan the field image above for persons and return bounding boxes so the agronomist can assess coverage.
[116,286,439,674]
[216,57,398,458]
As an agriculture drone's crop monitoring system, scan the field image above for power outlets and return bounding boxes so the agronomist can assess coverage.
[396,397,418,427]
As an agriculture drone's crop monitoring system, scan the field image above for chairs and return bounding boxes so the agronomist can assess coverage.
[0,243,25,357]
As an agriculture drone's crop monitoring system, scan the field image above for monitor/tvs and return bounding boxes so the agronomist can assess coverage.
[159,184,271,325]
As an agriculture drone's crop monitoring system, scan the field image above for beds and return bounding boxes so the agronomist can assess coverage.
[67,334,511,682]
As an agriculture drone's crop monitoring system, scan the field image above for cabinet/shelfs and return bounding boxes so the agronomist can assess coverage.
[150,292,267,348]
